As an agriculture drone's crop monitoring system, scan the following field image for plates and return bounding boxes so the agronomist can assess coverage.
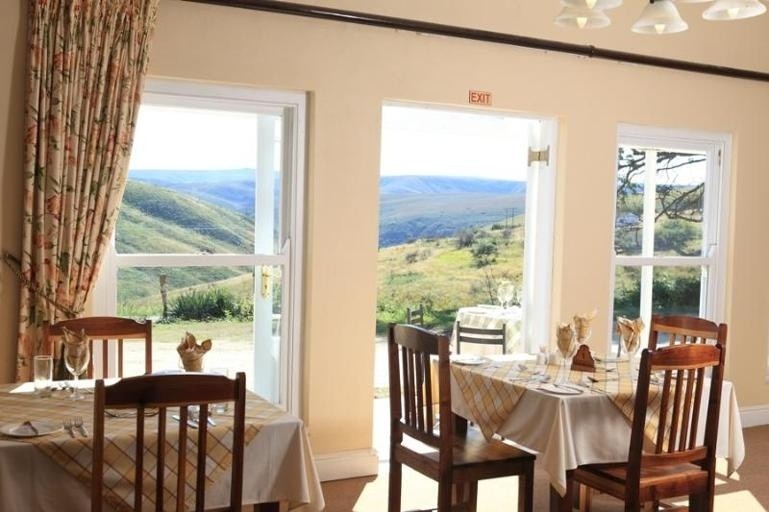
[451,353,485,365]
[1,421,63,436]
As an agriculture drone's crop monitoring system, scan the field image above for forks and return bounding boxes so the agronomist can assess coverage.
[64,415,88,438]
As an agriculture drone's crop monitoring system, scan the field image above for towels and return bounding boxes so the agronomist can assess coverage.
[61,322,92,372]
[175,329,214,372]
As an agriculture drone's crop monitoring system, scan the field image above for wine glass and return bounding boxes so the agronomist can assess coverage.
[65,346,90,400]
[531,325,701,398]
[466,281,521,317]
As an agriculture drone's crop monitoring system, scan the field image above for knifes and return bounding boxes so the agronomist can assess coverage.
[173,415,198,430]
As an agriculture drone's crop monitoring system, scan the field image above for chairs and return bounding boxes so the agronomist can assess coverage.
[569,343,726,510]
[646,315,728,362]
[40,314,156,382]
[388,303,538,511]
[89,368,248,511]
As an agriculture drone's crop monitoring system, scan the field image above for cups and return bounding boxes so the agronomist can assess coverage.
[34,355,52,399]
[179,357,205,372]
[210,403,228,412]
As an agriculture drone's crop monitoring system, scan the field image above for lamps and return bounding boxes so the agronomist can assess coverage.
[550,0,769,41]
